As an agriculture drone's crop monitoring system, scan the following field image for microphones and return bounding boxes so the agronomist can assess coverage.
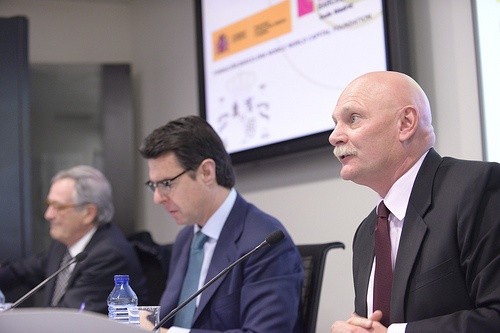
[153,230,286,332]
[10,252,88,309]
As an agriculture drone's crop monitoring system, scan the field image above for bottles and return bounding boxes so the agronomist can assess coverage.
[0,290,6,311]
[107,275,140,326]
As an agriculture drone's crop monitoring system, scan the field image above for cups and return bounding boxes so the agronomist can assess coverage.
[127,306,161,333]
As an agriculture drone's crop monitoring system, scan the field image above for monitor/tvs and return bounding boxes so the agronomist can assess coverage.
[193,0,392,167]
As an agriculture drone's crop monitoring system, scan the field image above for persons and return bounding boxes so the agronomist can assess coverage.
[328,71,500,333]
[0,165,138,317]
[140,115,304,333]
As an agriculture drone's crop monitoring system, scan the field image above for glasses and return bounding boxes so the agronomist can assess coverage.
[45,200,80,211]
[145,168,191,194]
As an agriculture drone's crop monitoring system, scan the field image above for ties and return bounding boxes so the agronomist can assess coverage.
[373,200,393,328]
[173,231,209,330]
[51,251,72,307]
